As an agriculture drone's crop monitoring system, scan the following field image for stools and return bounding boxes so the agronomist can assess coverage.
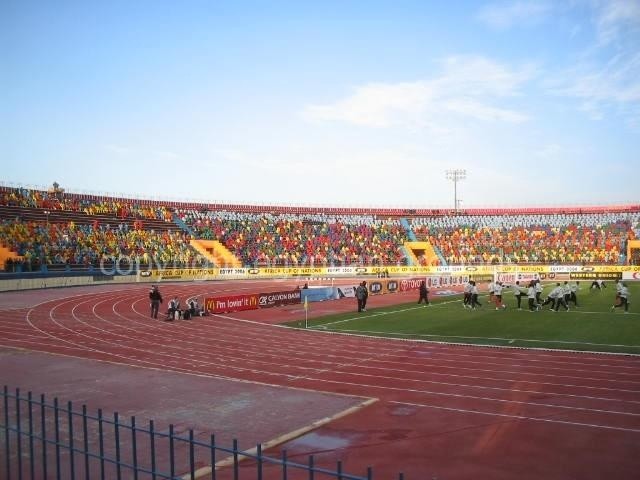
[1,207,205,275]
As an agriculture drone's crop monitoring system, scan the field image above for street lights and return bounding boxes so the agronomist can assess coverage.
[444,169,466,209]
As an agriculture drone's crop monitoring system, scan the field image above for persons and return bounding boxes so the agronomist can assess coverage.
[457,279,631,316]
[149,285,163,318]
[356,282,364,312]
[361,281,368,311]
[190,296,198,309]
[303,283,308,289]
[0,185,640,279]
[165,295,181,319]
[417,280,431,304]
